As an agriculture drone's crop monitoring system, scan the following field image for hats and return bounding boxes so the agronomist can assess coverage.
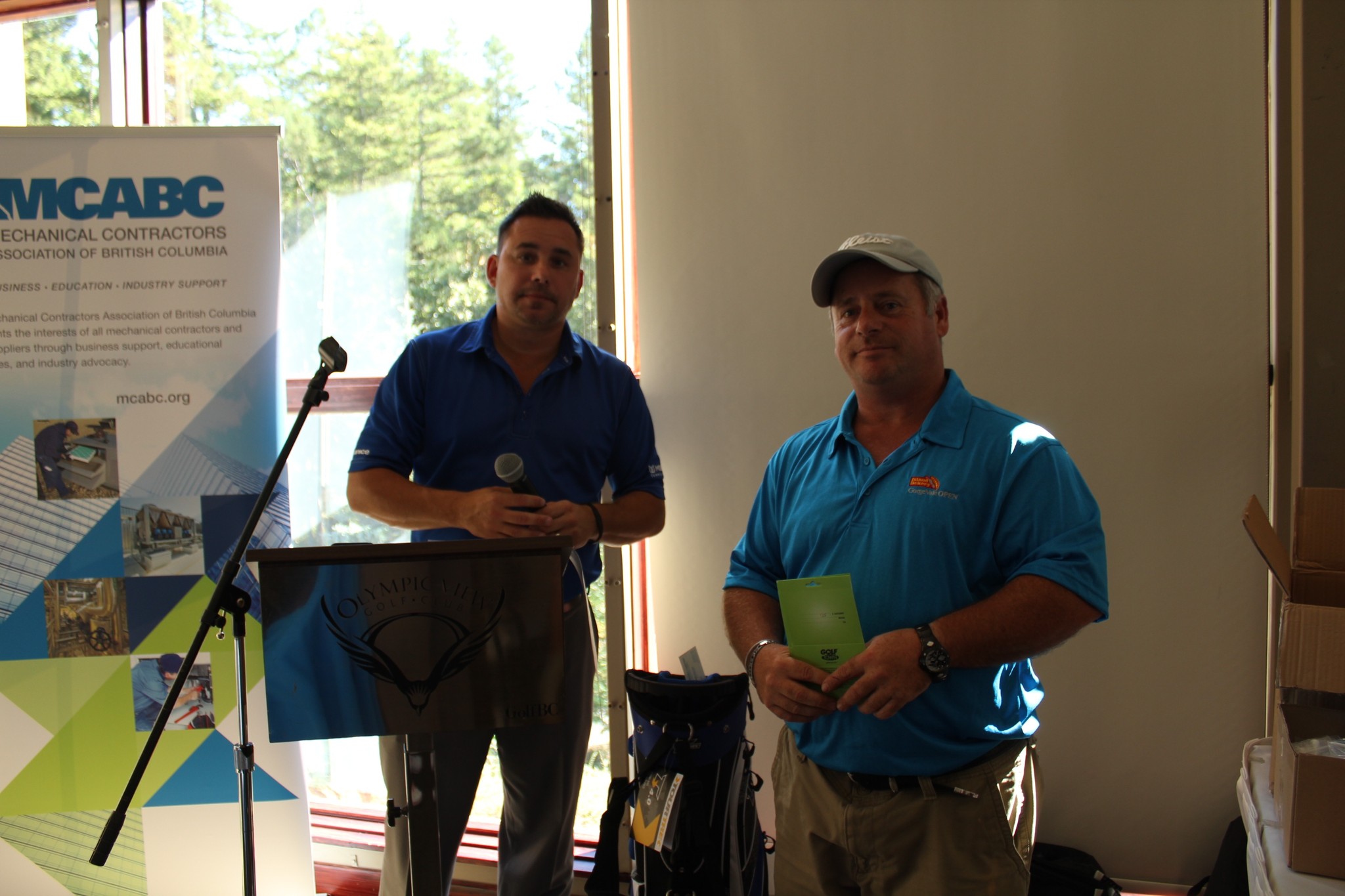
[809,233,944,308]
[67,420,79,436]
[160,654,184,672]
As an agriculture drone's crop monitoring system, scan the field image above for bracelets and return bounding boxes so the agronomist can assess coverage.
[584,502,604,542]
[743,636,778,686]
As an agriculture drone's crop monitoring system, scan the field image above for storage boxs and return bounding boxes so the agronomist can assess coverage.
[1242,486,1345,793]
[1276,703,1345,882]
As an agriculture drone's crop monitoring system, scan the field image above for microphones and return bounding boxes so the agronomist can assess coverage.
[495,452,578,559]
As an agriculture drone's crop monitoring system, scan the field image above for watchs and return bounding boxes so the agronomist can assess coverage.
[914,617,958,680]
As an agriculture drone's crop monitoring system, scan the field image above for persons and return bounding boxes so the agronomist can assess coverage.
[723,231,1111,896]
[347,191,666,895]
